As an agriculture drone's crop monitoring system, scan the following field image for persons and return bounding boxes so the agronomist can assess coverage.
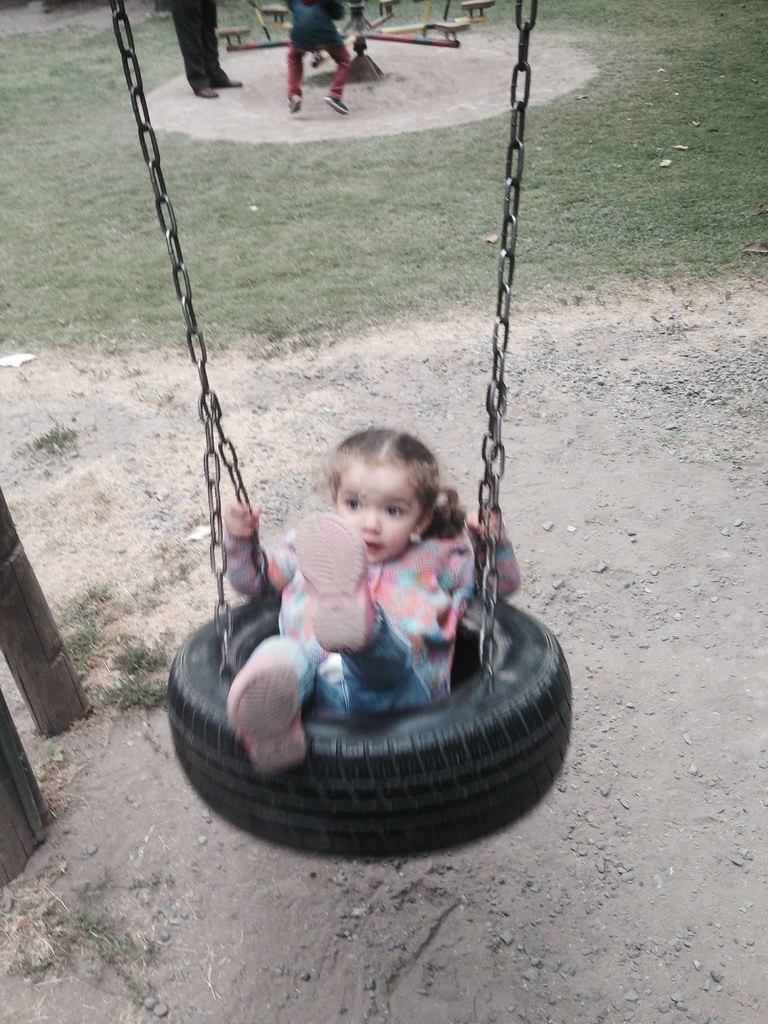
[286,0,352,115]
[218,429,520,774]
[172,0,242,98]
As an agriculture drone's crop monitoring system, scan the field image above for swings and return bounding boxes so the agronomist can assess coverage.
[109,0,576,867]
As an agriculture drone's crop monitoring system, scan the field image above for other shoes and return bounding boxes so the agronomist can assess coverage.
[296,514,375,655]
[324,96,349,114]
[288,95,302,113]
[195,86,218,98]
[219,78,242,87]
[227,661,305,773]
